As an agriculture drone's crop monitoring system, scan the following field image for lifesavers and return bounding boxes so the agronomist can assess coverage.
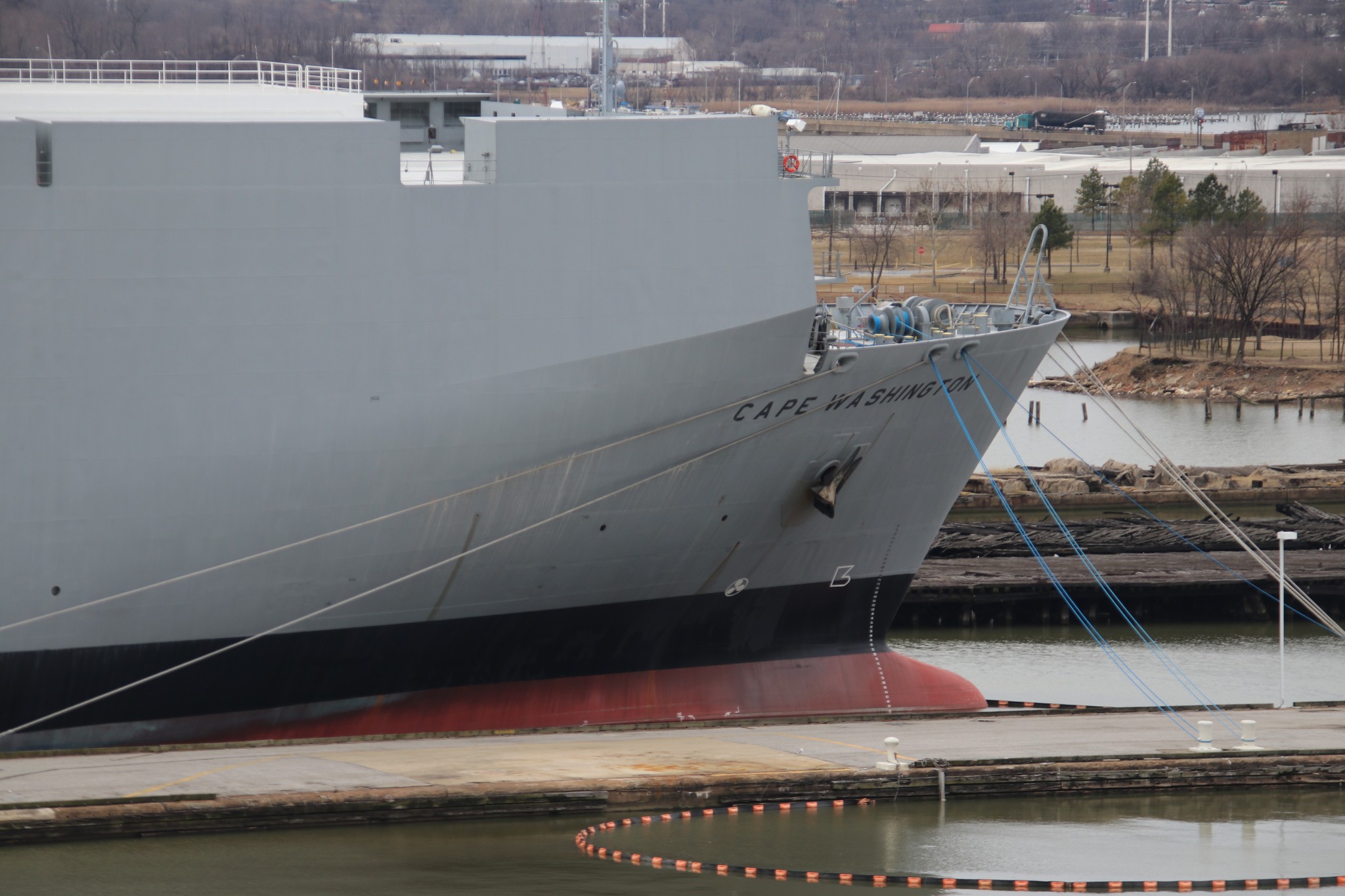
[783,154,799,172]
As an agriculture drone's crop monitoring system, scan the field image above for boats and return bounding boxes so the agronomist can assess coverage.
[0,0,1070,753]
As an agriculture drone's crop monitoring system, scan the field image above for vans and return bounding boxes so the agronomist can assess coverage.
[513,98,520,104]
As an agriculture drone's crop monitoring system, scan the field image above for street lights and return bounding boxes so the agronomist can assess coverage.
[34,47,49,78]
[891,68,912,84]
[291,56,305,69]
[818,71,829,121]
[547,57,551,80]
[164,51,177,80]
[575,57,579,78]
[562,64,566,85]
[231,54,245,84]
[874,70,887,115]
[101,50,114,82]
[1273,530,1298,709]
[967,76,982,126]
[1122,82,1138,131]
[533,63,536,82]
[785,61,794,81]
[1025,76,1038,112]
[1182,80,1193,134]
[636,63,640,111]
[762,77,777,92]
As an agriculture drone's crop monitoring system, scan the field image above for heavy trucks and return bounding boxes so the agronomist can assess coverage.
[1002,110,1110,135]
[749,104,791,122]
[576,99,599,109]
